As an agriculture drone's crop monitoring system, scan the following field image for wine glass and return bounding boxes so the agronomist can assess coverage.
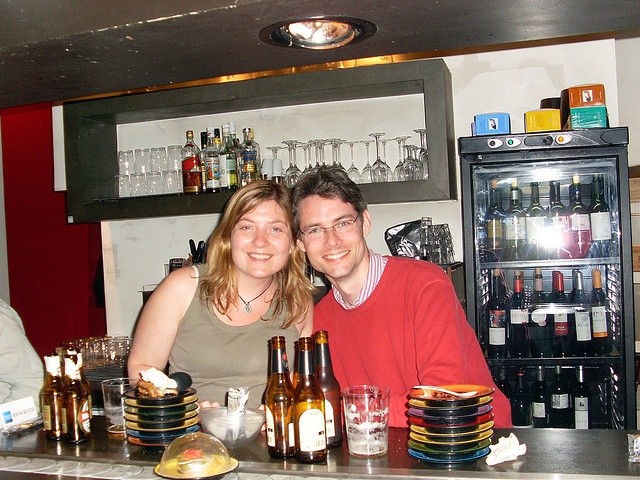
[379,139,393,183]
[266,146,281,160]
[411,148,423,181]
[282,140,309,187]
[414,129,428,180]
[309,140,326,172]
[392,138,406,182]
[325,138,346,171]
[402,145,417,181]
[360,141,378,184]
[369,133,388,183]
[398,136,413,181]
[343,141,361,185]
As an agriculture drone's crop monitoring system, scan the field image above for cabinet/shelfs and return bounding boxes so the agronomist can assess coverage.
[62,58,457,226]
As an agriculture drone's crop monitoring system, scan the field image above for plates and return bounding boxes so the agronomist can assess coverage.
[125,416,200,436]
[154,455,239,480]
[409,429,494,451]
[126,425,200,444]
[406,448,491,469]
[127,436,169,452]
[408,404,492,425]
[410,384,495,407]
[124,411,198,429]
[124,394,198,413]
[124,404,198,421]
[409,396,494,416]
[123,387,197,406]
[407,438,492,460]
[409,412,494,434]
[410,420,495,442]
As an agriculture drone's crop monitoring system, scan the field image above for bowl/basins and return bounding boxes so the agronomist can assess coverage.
[199,407,265,449]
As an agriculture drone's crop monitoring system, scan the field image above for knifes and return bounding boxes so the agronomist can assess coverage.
[189,238,206,264]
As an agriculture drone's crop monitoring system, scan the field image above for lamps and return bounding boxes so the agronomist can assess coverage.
[259,15,376,50]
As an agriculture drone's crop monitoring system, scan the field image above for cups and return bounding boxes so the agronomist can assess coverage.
[135,148,151,174]
[433,223,455,265]
[162,171,178,194]
[146,172,163,195]
[69,336,133,368]
[165,145,183,171]
[101,378,137,439]
[273,158,283,184]
[151,147,166,172]
[178,169,183,193]
[130,174,146,197]
[119,150,134,175]
[343,384,390,460]
[261,158,273,181]
[114,176,130,197]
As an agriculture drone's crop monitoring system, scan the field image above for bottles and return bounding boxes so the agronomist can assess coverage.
[291,337,327,463]
[550,364,570,429]
[315,330,342,449]
[485,180,506,262]
[199,132,208,194]
[264,336,296,459]
[570,365,592,429]
[487,268,514,359]
[262,339,271,404]
[588,175,614,258]
[531,366,549,428]
[504,178,526,261]
[530,268,548,357]
[61,354,90,445]
[228,122,239,143]
[292,341,299,390]
[67,348,94,430]
[213,128,221,148]
[548,271,573,356]
[587,269,612,354]
[509,271,531,358]
[182,130,202,195]
[205,127,220,194]
[526,182,546,260]
[420,218,433,260]
[568,269,594,356]
[546,180,568,259]
[39,352,64,442]
[568,176,592,258]
[236,127,263,189]
[218,125,238,192]
[511,365,532,426]
[497,366,513,400]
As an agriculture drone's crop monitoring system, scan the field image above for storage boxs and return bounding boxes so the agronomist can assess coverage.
[470,112,511,136]
[569,106,607,129]
[566,84,605,106]
[523,109,562,133]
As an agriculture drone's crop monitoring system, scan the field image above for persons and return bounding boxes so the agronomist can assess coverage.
[0,300,44,416]
[127,180,320,433]
[288,167,515,430]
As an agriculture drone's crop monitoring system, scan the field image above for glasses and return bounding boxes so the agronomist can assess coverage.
[299,212,360,240]
